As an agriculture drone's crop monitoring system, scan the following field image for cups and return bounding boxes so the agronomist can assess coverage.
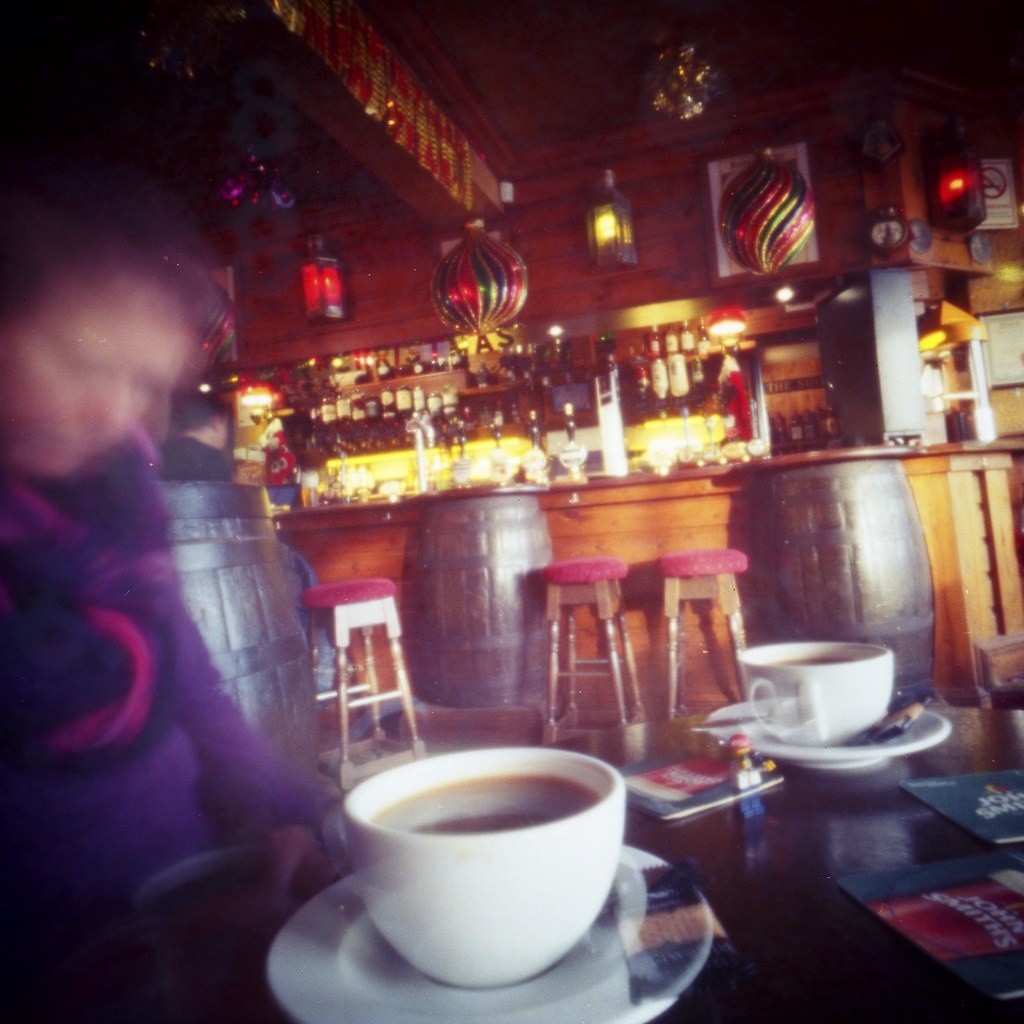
[342,751,627,990]
[737,640,895,749]
[301,473,319,508]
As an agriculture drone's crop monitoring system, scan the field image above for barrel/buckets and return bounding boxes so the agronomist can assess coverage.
[402,493,554,709]
[151,477,320,792]
[727,462,936,715]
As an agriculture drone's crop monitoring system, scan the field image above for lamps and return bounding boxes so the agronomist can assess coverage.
[709,311,744,336]
[927,126,990,233]
[584,167,637,271]
[299,234,347,325]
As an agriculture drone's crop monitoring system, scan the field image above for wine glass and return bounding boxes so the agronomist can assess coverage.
[451,439,588,489]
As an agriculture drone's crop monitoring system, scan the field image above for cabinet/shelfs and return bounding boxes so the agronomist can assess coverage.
[272,339,772,513]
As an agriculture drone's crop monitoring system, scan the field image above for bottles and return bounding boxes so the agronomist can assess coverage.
[456,403,582,452]
[649,316,710,358]
[319,384,458,425]
[430,354,440,372]
[768,406,840,445]
[477,359,491,387]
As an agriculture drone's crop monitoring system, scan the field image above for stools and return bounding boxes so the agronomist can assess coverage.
[662,549,748,722]
[299,578,427,792]
[539,558,648,749]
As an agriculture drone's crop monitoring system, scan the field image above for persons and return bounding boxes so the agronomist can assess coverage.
[0,194,340,1023]
[156,392,233,482]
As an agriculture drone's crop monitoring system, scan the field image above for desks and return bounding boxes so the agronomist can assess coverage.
[259,707,1024,1024]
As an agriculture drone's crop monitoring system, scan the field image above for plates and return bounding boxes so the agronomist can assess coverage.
[264,846,713,1024]
[706,701,953,770]
